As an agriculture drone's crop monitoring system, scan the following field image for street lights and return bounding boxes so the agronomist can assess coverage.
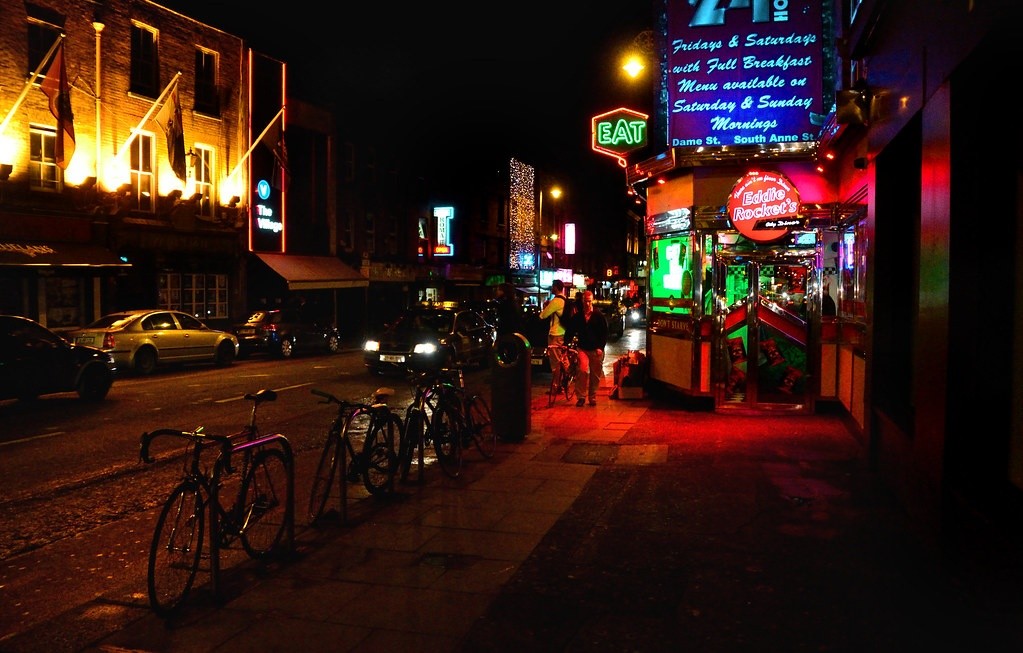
[550,186,562,267]
[626,33,656,131]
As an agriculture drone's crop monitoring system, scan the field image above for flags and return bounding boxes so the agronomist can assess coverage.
[156,84,186,183]
[261,113,291,194]
[39,46,76,171]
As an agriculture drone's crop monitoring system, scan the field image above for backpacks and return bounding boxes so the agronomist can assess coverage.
[551,295,580,327]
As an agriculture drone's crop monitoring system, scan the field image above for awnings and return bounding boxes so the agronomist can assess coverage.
[516,287,548,297]
[255,252,370,290]
[0,236,134,277]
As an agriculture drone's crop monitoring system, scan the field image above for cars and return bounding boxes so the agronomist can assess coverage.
[363,306,497,376]
[593,303,626,336]
[229,310,342,358]
[632,304,647,329]
[62,309,239,376]
[0,316,118,402]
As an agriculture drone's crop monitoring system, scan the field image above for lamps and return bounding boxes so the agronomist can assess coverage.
[622,29,667,78]
[117,184,132,197]
[190,193,203,201]
[0,164,12,181]
[168,190,182,202]
[228,196,240,208]
[76,176,97,190]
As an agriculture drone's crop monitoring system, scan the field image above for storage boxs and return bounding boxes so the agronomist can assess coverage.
[618,385,643,399]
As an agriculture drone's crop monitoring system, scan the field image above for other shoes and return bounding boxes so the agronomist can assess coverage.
[589,399,598,406]
[576,398,586,406]
[545,387,566,395]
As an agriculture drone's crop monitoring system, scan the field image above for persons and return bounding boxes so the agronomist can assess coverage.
[539,279,571,395]
[563,290,610,406]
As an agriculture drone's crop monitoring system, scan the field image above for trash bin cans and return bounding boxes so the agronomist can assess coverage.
[490,333,532,437]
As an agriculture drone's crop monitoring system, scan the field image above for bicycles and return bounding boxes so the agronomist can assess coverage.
[398,368,497,482]
[140,389,294,617]
[546,339,579,406]
[308,387,406,528]
